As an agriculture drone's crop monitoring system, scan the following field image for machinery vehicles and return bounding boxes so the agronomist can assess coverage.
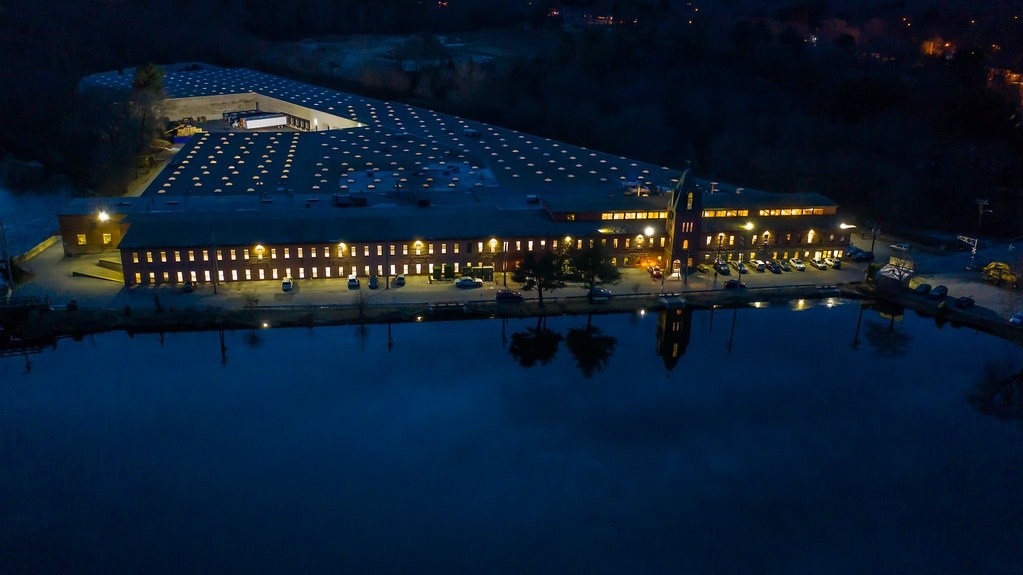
[981,261,1020,289]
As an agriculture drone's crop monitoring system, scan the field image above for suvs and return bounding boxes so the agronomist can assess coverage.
[587,287,612,299]
[749,260,765,271]
[347,275,358,288]
[714,259,730,275]
[396,274,405,286]
[789,258,806,271]
[824,258,841,269]
[368,275,379,288]
[281,277,293,289]
[890,243,910,252]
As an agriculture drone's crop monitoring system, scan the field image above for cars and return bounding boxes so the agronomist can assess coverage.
[184,281,194,292]
[647,265,663,278]
[810,258,826,270]
[454,277,483,288]
[496,290,524,302]
[766,259,791,273]
[724,280,746,289]
[731,261,747,273]
[914,284,1023,324]
[847,246,874,262]
